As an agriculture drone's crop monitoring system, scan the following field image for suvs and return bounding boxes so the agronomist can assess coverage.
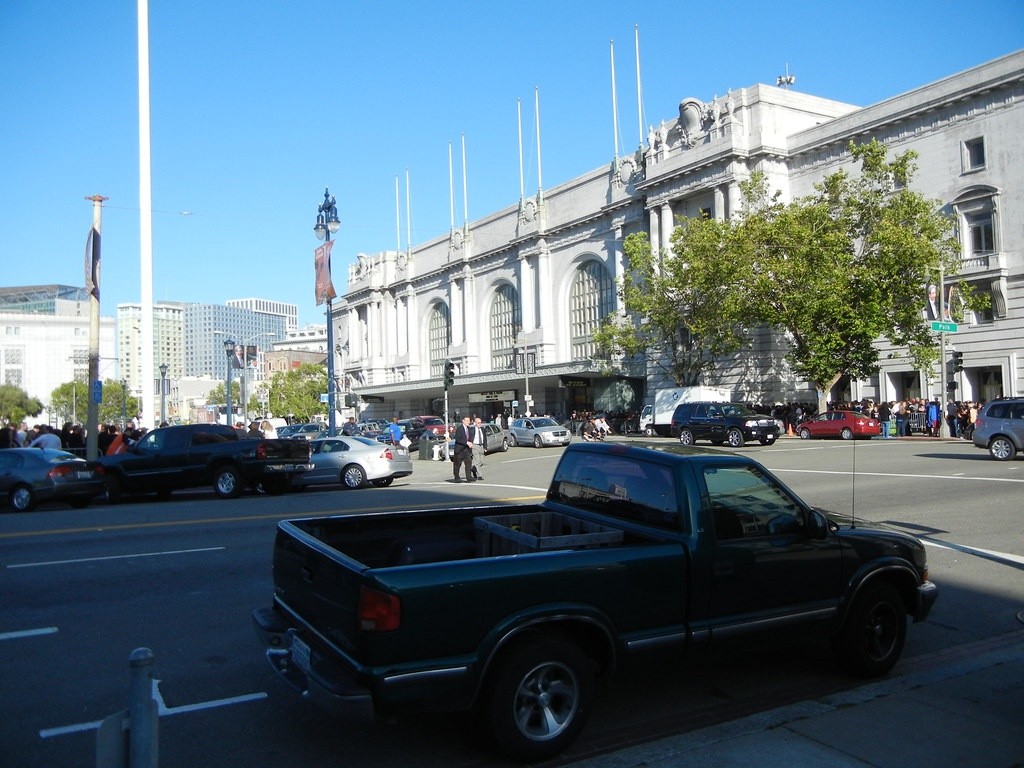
[972,396,1024,461]
[671,402,780,448]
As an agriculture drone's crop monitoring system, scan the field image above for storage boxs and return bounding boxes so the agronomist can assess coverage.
[473,511,624,558]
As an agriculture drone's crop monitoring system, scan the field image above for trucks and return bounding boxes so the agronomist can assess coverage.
[638,385,731,437]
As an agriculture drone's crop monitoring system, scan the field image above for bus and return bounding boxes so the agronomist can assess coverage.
[189,404,245,428]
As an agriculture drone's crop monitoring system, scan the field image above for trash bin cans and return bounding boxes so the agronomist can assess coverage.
[419,430,439,460]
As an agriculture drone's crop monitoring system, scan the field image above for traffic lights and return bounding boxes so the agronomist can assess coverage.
[953,351,964,374]
[948,381,957,390]
[444,362,454,386]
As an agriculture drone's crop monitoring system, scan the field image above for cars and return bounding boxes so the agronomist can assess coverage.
[254,416,456,452]
[252,436,413,495]
[796,411,880,440]
[507,417,572,448]
[0,448,108,512]
[439,424,509,462]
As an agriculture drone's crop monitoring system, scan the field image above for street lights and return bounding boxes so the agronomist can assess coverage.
[213,330,276,432]
[313,186,341,437]
[48,401,68,430]
[159,363,168,423]
[254,386,270,413]
[120,377,127,431]
[224,336,235,426]
[923,264,950,438]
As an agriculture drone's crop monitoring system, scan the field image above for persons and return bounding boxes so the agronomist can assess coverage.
[581,413,612,442]
[878,402,892,439]
[926,402,940,436]
[232,345,257,369]
[507,413,557,429]
[927,285,941,319]
[747,401,819,432]
[248,421,278,439]
[490,414,501,427]
[570,406,644,432]
[96,422,168,456]
[894,406,910,436]
[826,397,942,430]
[390,417,404,446]
[1,421,86,455]
[453,415,488,482]
[342,416,362,436]
[946,398,983,439]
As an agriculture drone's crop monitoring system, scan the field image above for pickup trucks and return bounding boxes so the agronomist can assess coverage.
[252,442,939,757]
[94,424,315,499]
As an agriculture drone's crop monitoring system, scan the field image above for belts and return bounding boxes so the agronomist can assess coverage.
[475,444,484,445]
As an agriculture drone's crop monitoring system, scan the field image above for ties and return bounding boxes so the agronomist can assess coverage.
[478,427,482,446]
[465,427,469,441]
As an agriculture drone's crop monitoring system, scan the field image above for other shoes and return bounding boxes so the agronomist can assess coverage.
[960,436,964,440]
[478,477,483,480]
[473,471,477,478]
[467,477,477,483]
[455,477,461,483]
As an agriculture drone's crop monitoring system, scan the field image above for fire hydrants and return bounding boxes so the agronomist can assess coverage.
[432,445,441,460]
